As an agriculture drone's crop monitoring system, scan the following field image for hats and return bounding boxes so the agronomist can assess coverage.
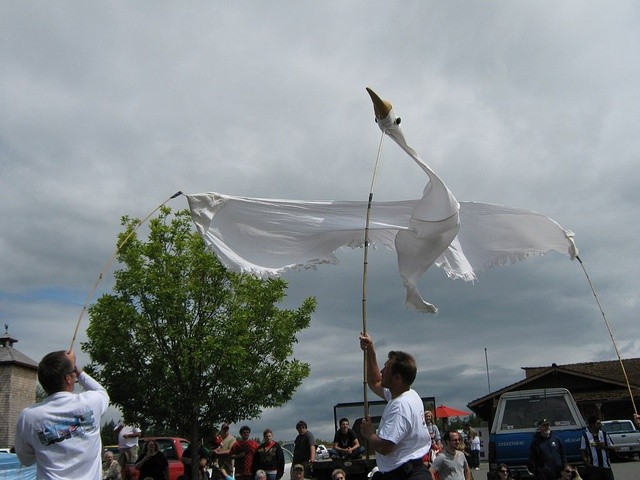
[538,419,550,426]
[222,423,229,429]
[294,464,304,470]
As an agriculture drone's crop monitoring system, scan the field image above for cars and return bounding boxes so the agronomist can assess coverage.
[279,447,295,480]
[281,443,324,476]
[597,419,640,463]
[119,436,191,480]
[318,444,330,459]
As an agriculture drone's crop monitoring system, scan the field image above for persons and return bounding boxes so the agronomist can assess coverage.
[495,463,516,480]
[329,417,365,461]
[134,439,171,480]
[290,420,318,480]
[118,424,142,464]
[527,417,567,480]
[211,423,237,480]
[331,468,346,480]
[180,437,211,480]
[228,426,259,480]
[579,415,618,480]
[464,423,477,439]
[293,464,311,480]
[14,349,111,480]
[101,450,123,480]
[255,470,266,480]
[559,463,583,480]
[251,429,284,480]
[422,440,441,480]
[429,430,471,480]
[424,410,442,445]
[634,414,640,428]
[469,431,481,471]
[458,433,470,456]
[358,330,433,480]
[212,462,234,479]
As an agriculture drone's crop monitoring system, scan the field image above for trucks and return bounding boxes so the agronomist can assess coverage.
[308,397,437,480]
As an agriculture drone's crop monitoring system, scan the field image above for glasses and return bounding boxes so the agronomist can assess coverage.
[450,439,460,441]
[67,366,77,374]
[499,470,509,473]
[563,470,574,474]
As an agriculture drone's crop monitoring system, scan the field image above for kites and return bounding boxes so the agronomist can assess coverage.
[184,85,579,316]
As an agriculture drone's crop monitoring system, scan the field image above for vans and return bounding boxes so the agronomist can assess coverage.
[487,388,590,478]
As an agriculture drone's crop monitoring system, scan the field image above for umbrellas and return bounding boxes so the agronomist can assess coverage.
[429,404,472,433]
